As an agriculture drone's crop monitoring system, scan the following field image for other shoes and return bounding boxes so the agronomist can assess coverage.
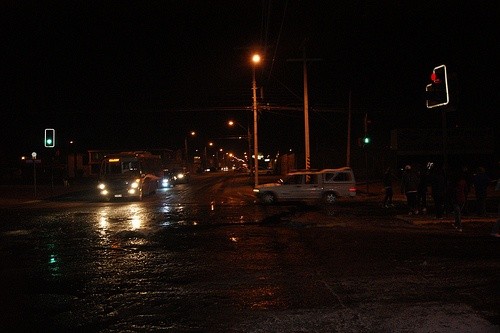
[414,210,419,215]
[391,204,394,207]
[383,204,388,208]
[408,211,413,216]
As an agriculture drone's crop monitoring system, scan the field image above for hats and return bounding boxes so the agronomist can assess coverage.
[404,165,412,174]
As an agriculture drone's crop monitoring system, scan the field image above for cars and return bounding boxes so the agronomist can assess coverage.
[234,166,274,177]
[171,170,192,187]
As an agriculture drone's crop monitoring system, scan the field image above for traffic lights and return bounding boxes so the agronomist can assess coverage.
[426,65,449,109]
[44,128,56,148]
[365,136,371,144]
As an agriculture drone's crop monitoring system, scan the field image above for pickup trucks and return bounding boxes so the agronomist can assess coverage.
[253,166,357,207]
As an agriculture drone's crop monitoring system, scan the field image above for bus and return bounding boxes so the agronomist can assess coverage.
[97,151,163,200]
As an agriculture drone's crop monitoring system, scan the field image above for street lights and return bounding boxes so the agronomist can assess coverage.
[228,120,253,177]
[184,131,195,159]
[249,52,262,187]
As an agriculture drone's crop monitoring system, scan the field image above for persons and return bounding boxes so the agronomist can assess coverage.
[382,165,394,208]
[473,165,490,217]
[399,164,427,217]
[429,160,469,233]
